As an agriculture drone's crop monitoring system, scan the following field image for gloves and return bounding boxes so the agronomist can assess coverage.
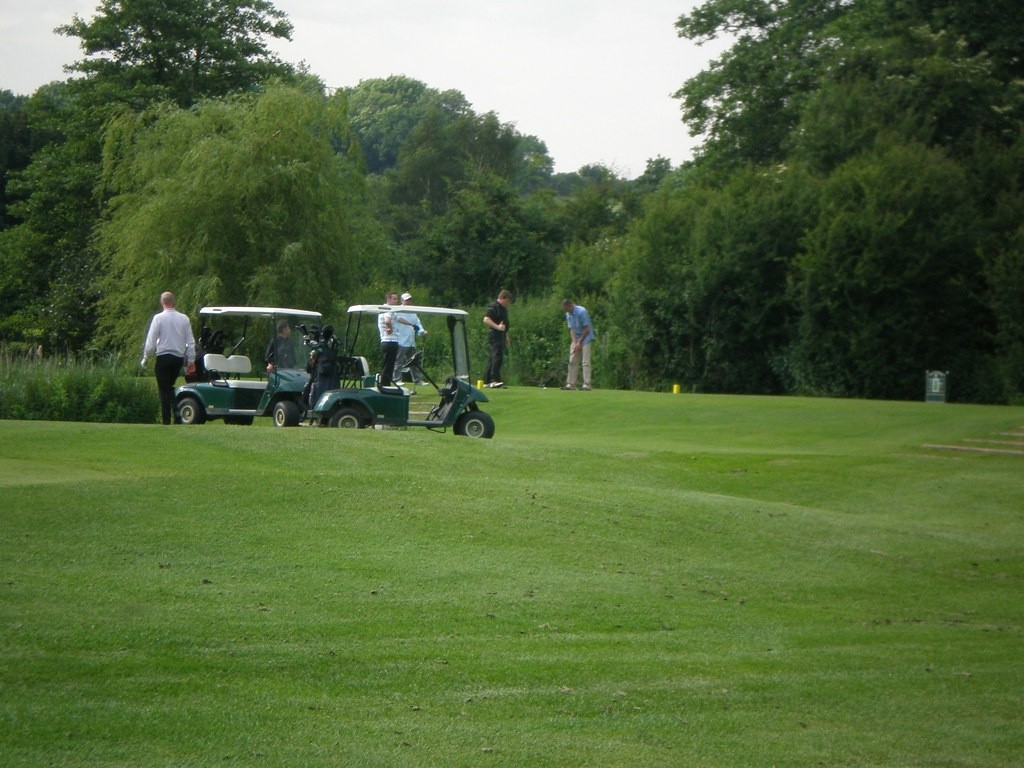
[141,355,149,369]
[414,325,420,332]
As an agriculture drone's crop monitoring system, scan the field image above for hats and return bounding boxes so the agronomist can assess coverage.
[401,293,411,301]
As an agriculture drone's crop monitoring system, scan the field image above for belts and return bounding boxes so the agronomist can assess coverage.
[381,340,397,343]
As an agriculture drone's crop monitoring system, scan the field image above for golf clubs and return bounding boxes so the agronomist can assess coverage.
[500,332,508,388]
[421,335,426,367]
[538,351,575,388]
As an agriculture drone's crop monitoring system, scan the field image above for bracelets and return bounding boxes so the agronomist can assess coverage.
[144,356,149,360]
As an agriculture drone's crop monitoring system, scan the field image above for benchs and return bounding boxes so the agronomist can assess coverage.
[353,356,411,396]
[204,353,269,390]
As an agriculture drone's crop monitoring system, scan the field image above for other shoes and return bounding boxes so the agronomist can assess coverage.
[395,382,405,386]
[484,380,504,388]
[579,384,592,391]
[561,383,576,390]
[175,418,182,424]
[422,383,429,386]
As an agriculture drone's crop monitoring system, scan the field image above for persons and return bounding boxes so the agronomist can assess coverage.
[483,289,514,388]
[561,299,594,391]
[140,292,196,425]
[264,320,297,381]
[378,292,429,386]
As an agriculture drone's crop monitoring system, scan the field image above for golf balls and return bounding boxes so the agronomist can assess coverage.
[543,387,546,390]
